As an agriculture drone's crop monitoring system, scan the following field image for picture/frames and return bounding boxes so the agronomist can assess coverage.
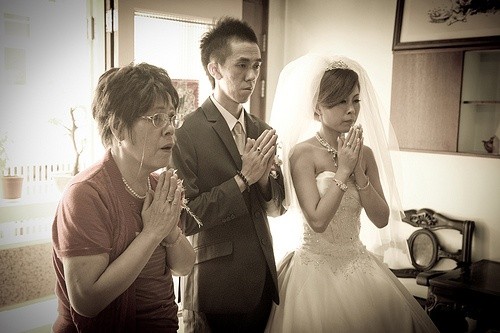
[392,0,500,50]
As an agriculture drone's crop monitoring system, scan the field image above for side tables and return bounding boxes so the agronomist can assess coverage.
[429,258,500,333]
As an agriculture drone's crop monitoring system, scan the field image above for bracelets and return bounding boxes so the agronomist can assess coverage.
[332,179,347,192]
[356,176,370,190]
[161,225,182,248]
[236,169,251,192]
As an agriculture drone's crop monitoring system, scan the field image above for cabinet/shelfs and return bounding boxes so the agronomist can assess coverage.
[388,48,500,159]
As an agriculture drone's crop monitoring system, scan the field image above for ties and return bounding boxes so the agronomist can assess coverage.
[233,123,246,161]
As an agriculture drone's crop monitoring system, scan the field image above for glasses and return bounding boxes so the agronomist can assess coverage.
[140,112,185,130]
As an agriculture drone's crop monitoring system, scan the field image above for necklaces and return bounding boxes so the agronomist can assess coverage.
[122,176,152,200]
[315,132,338,167]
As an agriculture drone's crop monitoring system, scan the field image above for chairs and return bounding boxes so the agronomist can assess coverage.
[389,209,475,333]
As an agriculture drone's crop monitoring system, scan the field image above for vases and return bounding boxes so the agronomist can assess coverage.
[4,175,23,199]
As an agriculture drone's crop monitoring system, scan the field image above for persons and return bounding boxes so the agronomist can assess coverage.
[167,17,289,333]
[50,62,196,333]
[265,51,438,333]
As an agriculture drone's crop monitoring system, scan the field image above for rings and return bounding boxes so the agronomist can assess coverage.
[347,143,351,147]
[166,197,173,203]
[261,153,265,157]
[257,147,261,152]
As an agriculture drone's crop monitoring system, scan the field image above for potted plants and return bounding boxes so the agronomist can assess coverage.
[54,107,85,189]
